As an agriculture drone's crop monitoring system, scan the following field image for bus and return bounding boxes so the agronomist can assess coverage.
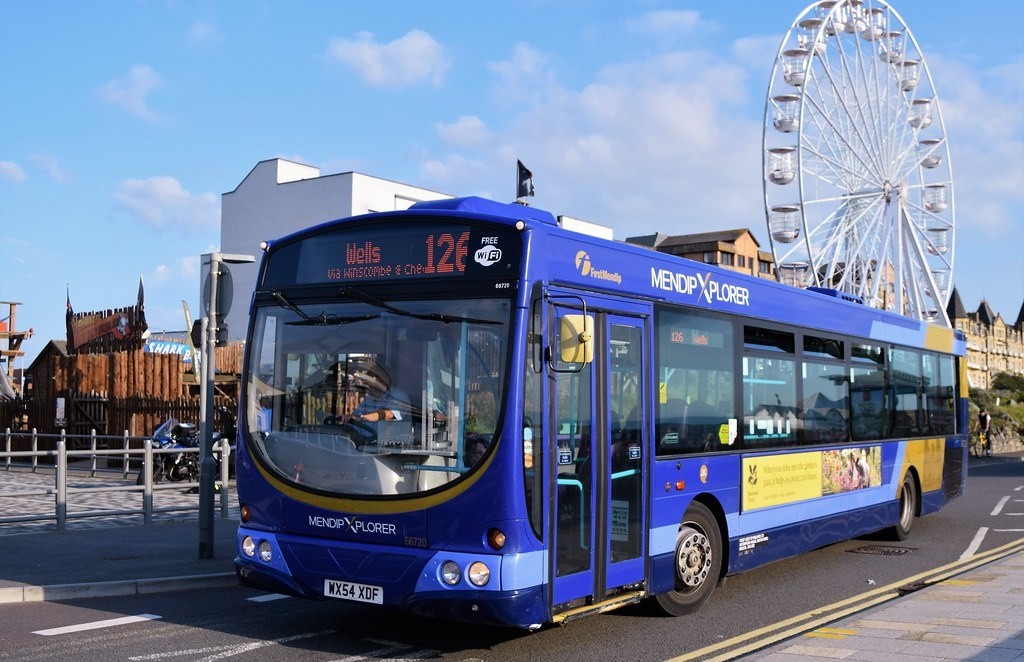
[234,195,970,632]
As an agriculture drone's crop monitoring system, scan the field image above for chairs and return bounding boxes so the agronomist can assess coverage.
[582,398,732,487]
[410,395,423,444]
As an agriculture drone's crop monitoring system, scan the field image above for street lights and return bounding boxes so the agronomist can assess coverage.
[197,253,256,559]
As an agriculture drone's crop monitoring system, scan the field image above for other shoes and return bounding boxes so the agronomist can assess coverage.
[988,450,990,453]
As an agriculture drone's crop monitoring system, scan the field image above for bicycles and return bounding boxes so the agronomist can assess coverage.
[972,428,994,459]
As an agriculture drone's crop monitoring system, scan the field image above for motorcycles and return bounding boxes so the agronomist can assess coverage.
[136,417,223,492]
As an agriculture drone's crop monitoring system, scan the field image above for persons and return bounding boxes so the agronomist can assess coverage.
[433,374,474,439]
[339,365,412,444]
[973,409,992,457]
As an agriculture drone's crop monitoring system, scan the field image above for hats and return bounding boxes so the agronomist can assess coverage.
[979,409,984,413]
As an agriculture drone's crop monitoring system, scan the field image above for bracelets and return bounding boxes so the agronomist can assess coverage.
[357,414,364,423]
